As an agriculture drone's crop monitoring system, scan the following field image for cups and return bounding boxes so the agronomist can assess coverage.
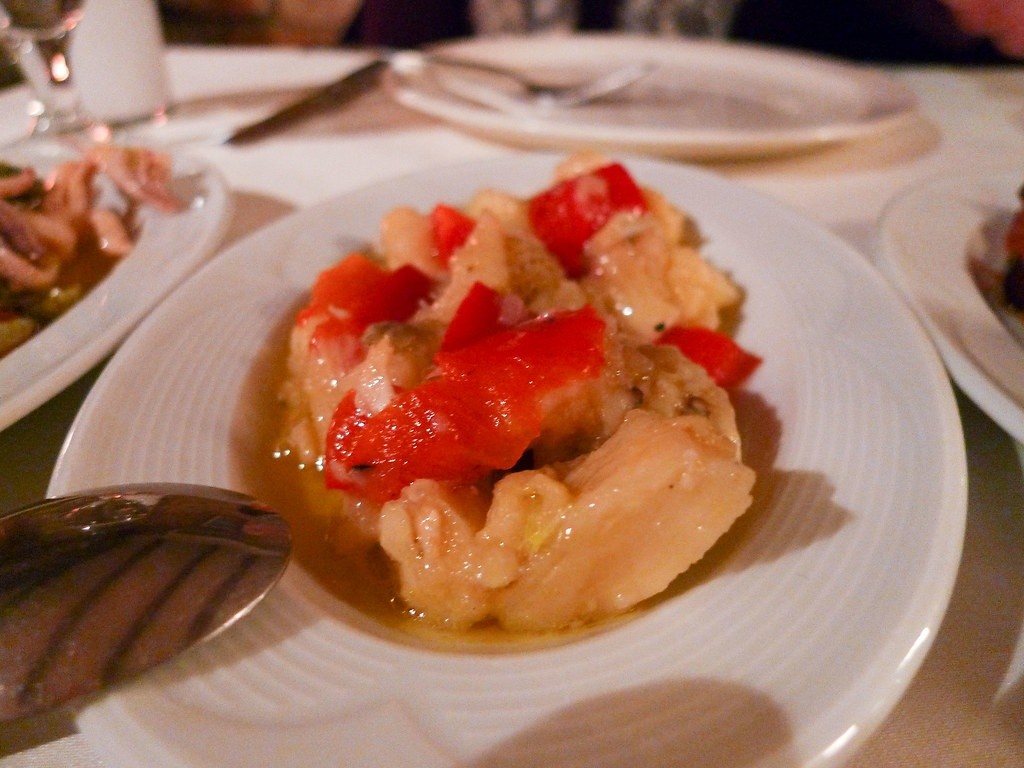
[7,1,174,133]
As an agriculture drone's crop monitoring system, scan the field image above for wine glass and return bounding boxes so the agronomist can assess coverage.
[0,0,114,151]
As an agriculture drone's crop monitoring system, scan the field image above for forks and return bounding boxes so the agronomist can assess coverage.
[424,55,653,109]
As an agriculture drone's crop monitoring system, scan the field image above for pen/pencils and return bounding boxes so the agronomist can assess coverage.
[224,60,386,146]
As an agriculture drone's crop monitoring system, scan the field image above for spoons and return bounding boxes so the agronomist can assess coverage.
[0,481,293,725]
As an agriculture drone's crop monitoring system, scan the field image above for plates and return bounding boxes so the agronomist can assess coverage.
[380,31,922,166]
[0,40,381,171]
[47,153,972,768]
[0,134,234,435]
[876,165,1024,442]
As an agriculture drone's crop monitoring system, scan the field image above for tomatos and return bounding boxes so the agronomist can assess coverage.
[295,163,761,502]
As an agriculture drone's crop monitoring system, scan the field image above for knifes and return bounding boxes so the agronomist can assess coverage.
[223,60,388,147]
